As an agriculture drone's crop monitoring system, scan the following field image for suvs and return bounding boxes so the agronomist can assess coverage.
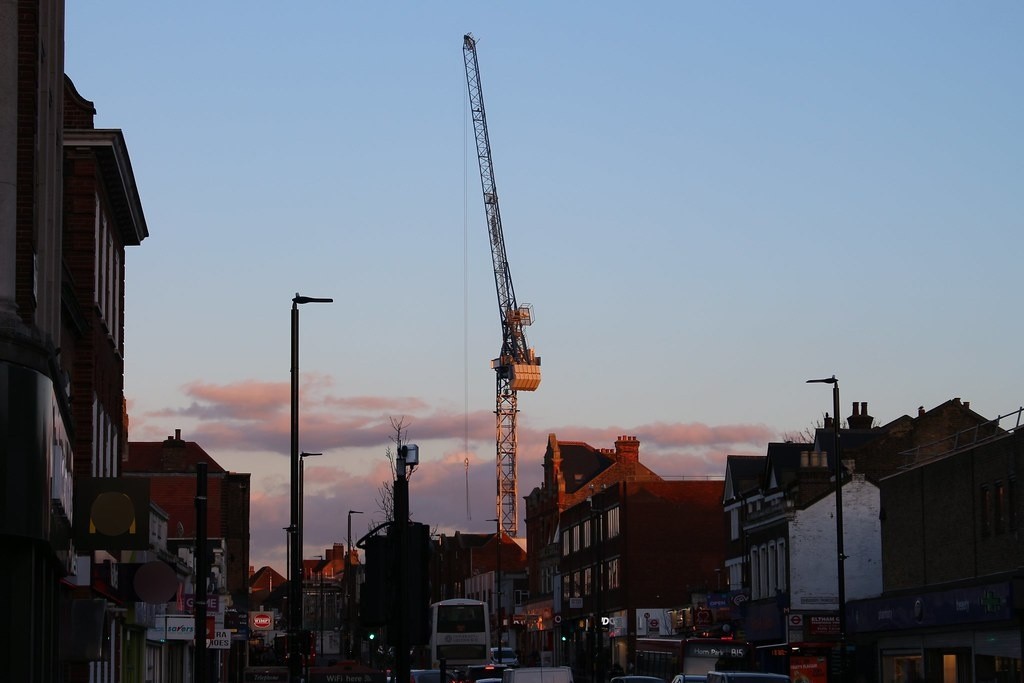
[707,671,791,683]
[466,663,509,683]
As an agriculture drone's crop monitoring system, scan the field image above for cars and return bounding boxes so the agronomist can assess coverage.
[492,647,518,666]
[671,674,707,683]
[609,675,668,683]
[409,669,461,683]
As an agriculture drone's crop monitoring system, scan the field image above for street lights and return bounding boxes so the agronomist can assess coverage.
[288,291,336,683]
[805,374,851,683]
[297,450,325,682]
[345,510,366,683]
[485,518,503,663]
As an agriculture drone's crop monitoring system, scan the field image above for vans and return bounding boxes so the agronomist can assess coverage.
[502,666,574,683]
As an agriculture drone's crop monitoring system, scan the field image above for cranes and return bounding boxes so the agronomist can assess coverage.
[461,32,544,540]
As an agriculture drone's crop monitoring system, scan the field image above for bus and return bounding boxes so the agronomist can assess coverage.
[634,636,750,675]
[424,597,493,683]
